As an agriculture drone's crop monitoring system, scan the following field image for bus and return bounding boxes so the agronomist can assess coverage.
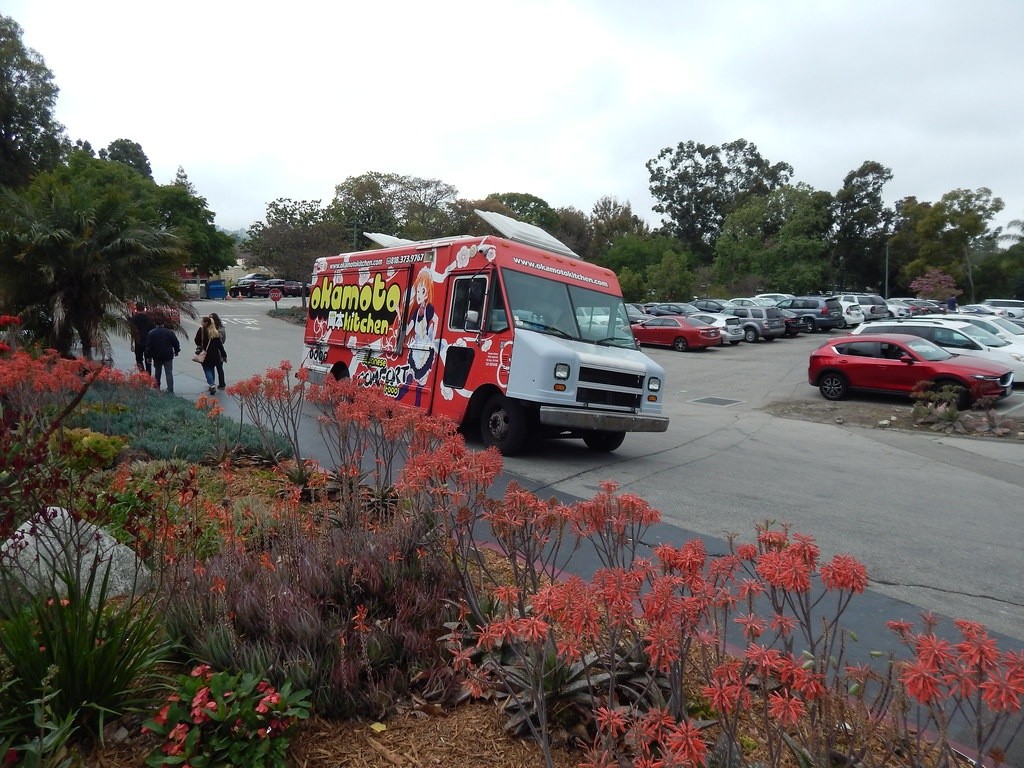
[779,308,809,337]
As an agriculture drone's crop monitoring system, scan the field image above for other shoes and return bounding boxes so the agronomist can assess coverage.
[217,385,225,390]
[208,387,211,391]
[210,387,216,395]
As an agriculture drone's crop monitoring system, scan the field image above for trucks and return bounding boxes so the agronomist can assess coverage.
[294,206,670,456]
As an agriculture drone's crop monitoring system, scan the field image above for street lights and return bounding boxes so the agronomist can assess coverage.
[347,219,363,252]
[884,242,888,300]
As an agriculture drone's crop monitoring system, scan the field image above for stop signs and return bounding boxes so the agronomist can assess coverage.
[269,288,282,301]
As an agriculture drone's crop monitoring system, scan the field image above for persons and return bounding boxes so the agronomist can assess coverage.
[144,318,180,391]
[948,294,959,311]
[194,317,228,395]
[249,281,256,296]
[129,302,155,377]
[209,313,227,390]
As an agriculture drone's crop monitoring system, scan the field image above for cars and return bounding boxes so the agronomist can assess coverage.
[238,272,311,298]
[885,297,1024,346]
[579,323,642,352]
[575,292,796,327]
[619,315,722,352]
[838,300,865,329]
[689,311,745,346]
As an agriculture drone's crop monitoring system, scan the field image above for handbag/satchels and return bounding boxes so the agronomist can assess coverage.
[192,349,207,363]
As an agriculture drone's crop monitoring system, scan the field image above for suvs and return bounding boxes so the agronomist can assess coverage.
[809,333,1015,413]
[831,291,890,321]
[848,317,1024,385]
[720,307,786,343]
[774,297,844,333]
